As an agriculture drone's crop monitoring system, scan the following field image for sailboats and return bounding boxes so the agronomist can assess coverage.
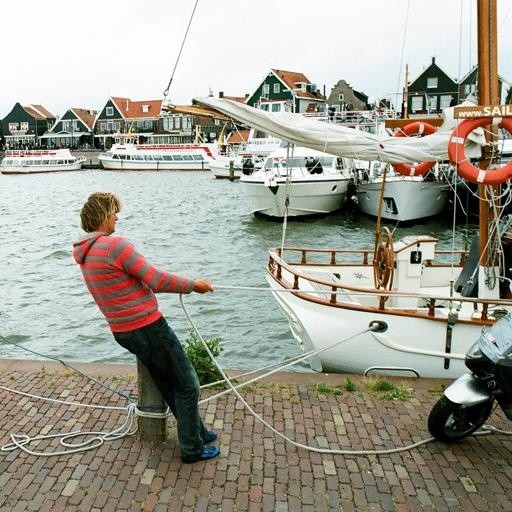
[265,1,512,379]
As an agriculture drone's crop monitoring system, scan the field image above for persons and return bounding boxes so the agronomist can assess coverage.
[70,190,220,464]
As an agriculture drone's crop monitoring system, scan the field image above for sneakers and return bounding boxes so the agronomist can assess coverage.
[203,431,217,444]
[181,446,220,463]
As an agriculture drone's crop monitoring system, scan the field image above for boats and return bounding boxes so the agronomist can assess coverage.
[0,147,89,173]
[98,140,215,170]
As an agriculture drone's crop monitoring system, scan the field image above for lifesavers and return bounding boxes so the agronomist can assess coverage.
[448,117,511,185]
[391,122,438,175]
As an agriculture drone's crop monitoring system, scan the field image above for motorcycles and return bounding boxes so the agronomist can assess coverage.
[426,276,511,443]
